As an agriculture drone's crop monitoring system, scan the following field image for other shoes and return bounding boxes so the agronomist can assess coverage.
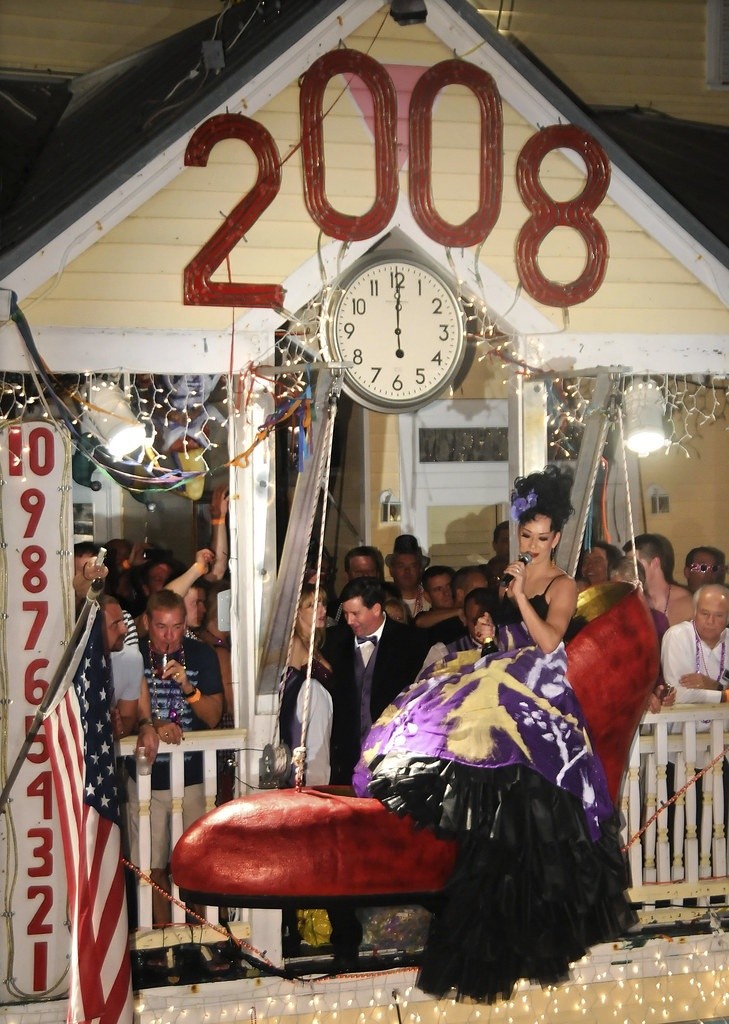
[200,949,231,976]
[329,947,360,973]
[221,956,247,975]
[144,950,170,976]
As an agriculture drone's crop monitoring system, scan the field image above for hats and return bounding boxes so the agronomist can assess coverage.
[384,534,431,570]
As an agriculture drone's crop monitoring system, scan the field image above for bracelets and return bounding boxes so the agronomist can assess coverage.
[210,517,225,526]
[181,684,197,700]
[185,687,201,703]
[715,680,724,691]
[83,561,90,581]
[192,562,205,576]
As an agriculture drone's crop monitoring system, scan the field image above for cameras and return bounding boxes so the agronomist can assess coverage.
[95,547,107,567]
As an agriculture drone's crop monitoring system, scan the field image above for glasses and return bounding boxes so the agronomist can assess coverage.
[689,564,720,574]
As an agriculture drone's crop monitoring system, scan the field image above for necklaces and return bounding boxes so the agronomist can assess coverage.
[663,582,671,615]
[693,619,711,678]
[148,638,186,724]
[691,622,725,682]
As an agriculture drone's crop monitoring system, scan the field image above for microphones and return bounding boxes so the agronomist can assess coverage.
[500,551,534,588]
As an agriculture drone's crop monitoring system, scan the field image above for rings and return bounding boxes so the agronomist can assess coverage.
[175,672,180,678]
[164,733,168,736]
[697,684,699,688]
[517,569,520,573]
[476,632,481,638]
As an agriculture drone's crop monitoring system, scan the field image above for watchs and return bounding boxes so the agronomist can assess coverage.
[138,718,154,728]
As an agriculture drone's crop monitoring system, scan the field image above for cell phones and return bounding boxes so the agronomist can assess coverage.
[659,682,671,704]
[722,669,729,683]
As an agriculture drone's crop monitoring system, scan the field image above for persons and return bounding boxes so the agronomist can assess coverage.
[460,465,578,1003]
[75,483,729,1005]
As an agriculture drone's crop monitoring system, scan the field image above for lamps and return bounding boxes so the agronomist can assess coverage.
[79,377,146,460]
[620,373,668,456]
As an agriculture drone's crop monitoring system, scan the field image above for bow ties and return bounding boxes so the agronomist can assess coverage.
[357,635,378,646]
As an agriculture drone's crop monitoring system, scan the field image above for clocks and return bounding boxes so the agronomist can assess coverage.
[318,249,469,414]
